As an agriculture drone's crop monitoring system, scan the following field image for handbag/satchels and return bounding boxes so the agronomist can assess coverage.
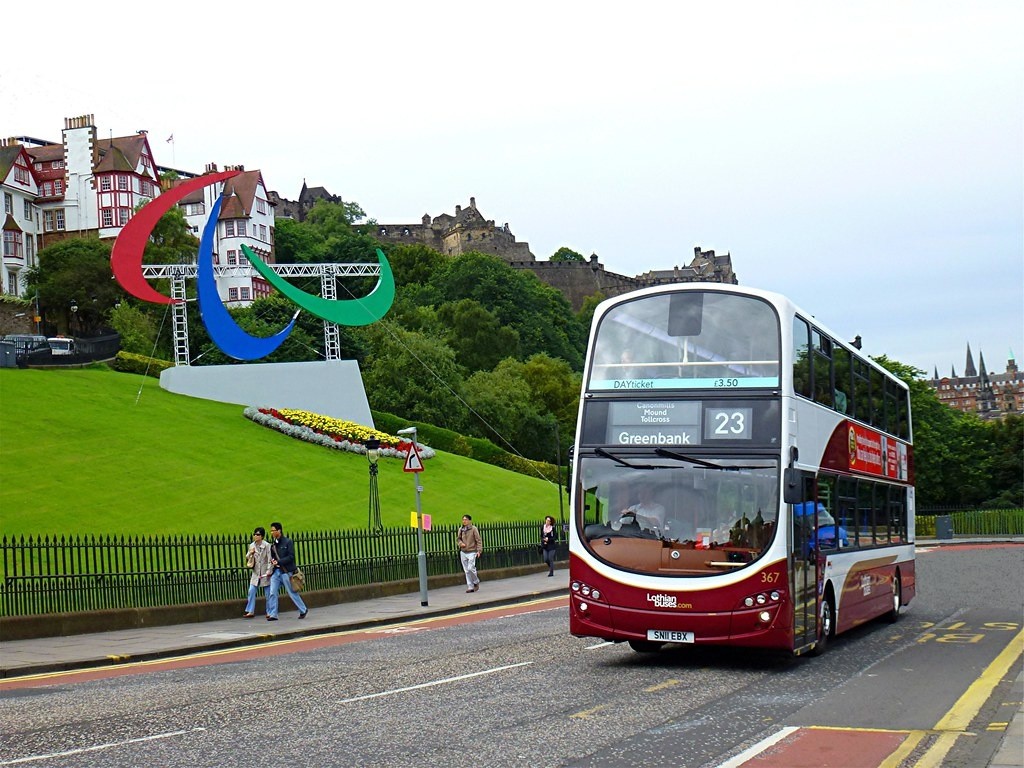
[247,553,255,569]
[537,544,543,555]
[457,547,461,561]
[289,567,306,593]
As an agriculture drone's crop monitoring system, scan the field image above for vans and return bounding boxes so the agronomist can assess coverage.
[787,501,849,565]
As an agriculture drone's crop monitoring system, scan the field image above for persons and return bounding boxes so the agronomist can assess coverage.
[456,515,482,593]
[540,516,558,577]
[610,350,648,380]
[620,486,667,536]
[834,389,847,412]
[242,528,273,618]
[267,522,308,621]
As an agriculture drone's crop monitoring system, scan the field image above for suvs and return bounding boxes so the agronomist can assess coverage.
[46,337,75,356]
[4,334,54,366]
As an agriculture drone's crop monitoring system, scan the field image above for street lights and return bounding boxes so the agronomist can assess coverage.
[397,426,429,607]
[70,298,78,337]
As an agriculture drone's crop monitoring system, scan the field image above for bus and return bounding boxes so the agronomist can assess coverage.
[564,285,918,656]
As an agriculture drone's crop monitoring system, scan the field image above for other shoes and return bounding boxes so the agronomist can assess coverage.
[474,582,480,591]
[298,608,309,619]
[267,617,278,621]
[466,589,474,593]
[548,573,553,577]
[266,613,270,618]
[242,614,255,618]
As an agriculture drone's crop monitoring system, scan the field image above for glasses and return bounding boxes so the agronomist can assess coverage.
[253,533,261,536]
[270,530,278,533]
